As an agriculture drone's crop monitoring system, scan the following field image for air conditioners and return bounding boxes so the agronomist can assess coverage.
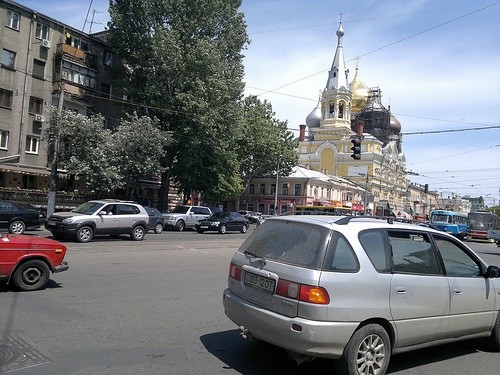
[41,39,51,49]
[34,113,44,122]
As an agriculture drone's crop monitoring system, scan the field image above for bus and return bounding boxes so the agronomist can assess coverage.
[428,208,471,239]
[466,209,500,242]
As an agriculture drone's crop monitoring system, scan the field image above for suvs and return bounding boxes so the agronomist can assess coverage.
[161,204,212,231]
[143,206,166,235]
[44,198,151,244]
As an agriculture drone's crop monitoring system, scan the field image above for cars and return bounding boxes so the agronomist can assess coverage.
[237,210,275,224]
[0,233,69,291]
[195,211,250,234]
[0,199,41,234]
[221,210,500,375]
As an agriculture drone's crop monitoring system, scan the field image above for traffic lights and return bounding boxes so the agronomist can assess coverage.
[350,137,362,161]
